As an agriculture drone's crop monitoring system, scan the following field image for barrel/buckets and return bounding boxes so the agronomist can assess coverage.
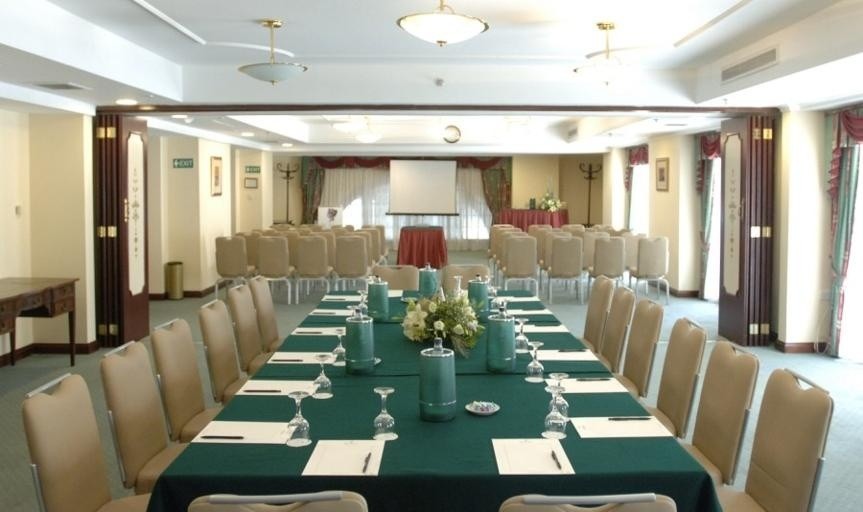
[419,348,456,423]
[345,316,376,375]
[486,314,517,373]
[468,279,489,323]
[418,268,438,302]
[367,281,390,323]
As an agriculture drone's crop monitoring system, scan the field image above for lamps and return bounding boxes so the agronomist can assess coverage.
[392,2,488,48]
[570,21,647,86]
[232,17,309,87]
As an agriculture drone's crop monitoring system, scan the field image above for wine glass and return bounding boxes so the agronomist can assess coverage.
[285,317,571,450]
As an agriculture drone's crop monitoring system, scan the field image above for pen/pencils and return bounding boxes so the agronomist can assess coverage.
[296,332,322,334]
[200,435,244,439]
[272,359,303,362]
[536,324,559,326]
[243,389,281,393]
[576,378,611,381]
[326,298,346,300]
[608,417,651,421]
[552,450,561,469]
[312,312,336,314]
[559,349,586,352]
[362,453,371,472]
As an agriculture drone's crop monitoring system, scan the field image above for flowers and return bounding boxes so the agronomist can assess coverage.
[538,191,566,213]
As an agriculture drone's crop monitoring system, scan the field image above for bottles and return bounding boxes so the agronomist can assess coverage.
[342,265,519,423]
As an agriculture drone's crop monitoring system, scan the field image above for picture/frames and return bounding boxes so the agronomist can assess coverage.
[209,155,222,197]
[654,156,670,192]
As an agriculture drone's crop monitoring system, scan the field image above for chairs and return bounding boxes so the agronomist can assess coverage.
[0,209,837,512]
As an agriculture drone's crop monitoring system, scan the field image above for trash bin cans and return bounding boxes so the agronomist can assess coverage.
[167,261,184,301]
